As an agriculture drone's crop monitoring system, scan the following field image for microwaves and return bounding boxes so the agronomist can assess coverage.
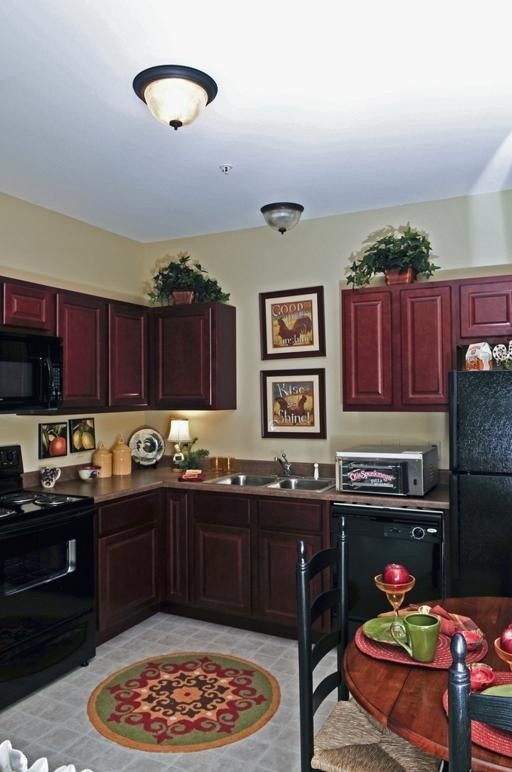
[335,443,440,496]
[0,335,65,408]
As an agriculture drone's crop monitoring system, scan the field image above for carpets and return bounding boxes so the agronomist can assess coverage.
[86,647,282,753]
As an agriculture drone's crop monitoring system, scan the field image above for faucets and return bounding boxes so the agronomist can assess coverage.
[274,453,292,477]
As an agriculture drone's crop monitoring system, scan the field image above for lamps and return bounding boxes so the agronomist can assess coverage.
[131,63,219,134]
[259,201,305,236]
[167,418,193,452]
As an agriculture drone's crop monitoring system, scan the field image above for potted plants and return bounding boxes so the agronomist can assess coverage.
[144,253,232,306]
[344,222,442,292]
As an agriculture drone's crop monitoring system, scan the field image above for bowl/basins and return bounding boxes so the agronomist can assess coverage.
[467,664,496,690]
[78,465,102,483]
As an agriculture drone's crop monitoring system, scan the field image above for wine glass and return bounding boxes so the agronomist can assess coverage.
[494,638,512,674]
[375,573,415,639]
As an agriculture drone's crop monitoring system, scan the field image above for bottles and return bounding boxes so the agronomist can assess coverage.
[92,431,130,481]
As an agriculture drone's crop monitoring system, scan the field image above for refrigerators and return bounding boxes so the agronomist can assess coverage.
[445,370,512,596]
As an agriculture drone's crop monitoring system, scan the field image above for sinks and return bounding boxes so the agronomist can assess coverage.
[263,477,336,495]
[213,473,280,489]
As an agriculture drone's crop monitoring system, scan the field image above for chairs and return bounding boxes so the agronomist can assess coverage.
[294,513,448,771]
[442,628,512,772]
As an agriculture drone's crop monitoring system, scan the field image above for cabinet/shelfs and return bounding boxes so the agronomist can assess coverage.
[341,281,453,412]
[95,488,168,640]
[167,487,330,635]
[453,274,512,345]
[149,303,238,412]
[58,289,150,413]
[1,276,56,336]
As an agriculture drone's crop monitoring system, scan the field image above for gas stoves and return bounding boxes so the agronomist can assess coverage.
[0,481,93,528]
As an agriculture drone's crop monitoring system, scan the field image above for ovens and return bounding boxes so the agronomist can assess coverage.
[329,502,445,644]
[0,515,98,707]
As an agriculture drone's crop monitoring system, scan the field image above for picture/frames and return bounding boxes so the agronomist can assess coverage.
[258,366,327,440]
[38,421,68,459]
[257,283,327,362]
[68,418,97,454]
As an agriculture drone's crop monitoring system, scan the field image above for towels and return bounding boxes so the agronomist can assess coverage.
[407,601,485,642]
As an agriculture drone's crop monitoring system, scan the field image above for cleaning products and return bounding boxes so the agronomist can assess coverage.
[93,434,132,478]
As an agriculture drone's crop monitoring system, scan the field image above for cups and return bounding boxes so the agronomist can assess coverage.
[40,464,61,489]
[210,455,236,474]
[390,614,441,663]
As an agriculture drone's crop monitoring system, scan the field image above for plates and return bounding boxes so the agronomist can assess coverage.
[482,683,512,698]
[363,615,416,645]
[129,426,165,466]
